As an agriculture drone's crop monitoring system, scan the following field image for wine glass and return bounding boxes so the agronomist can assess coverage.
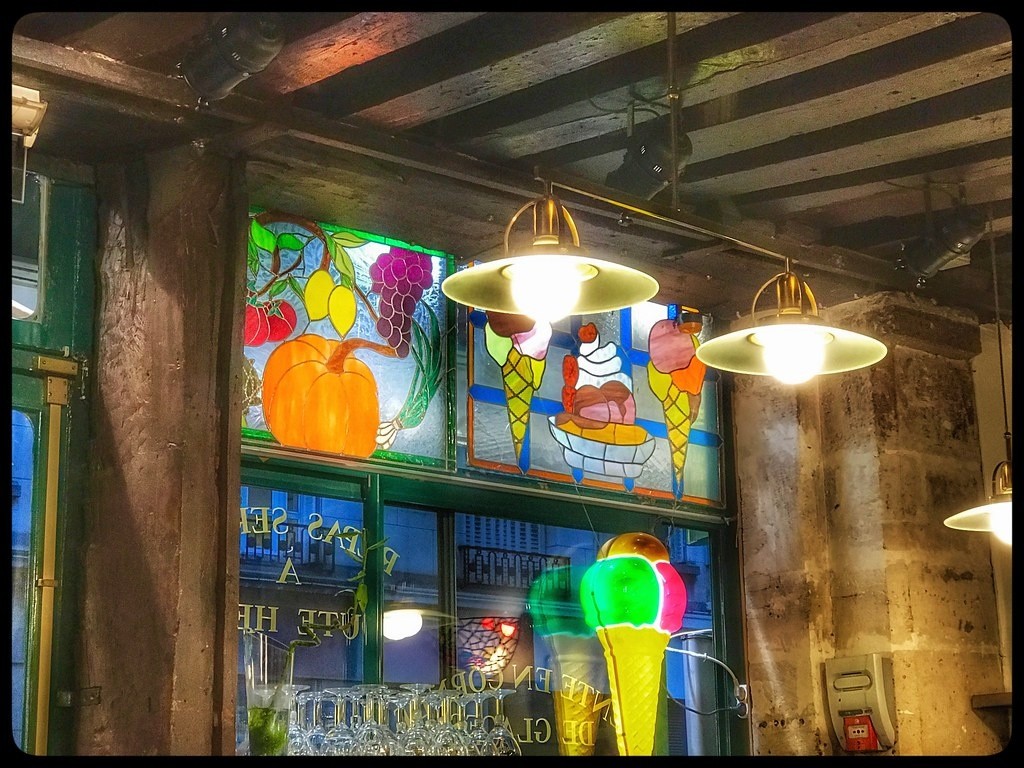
[237,684,523,756]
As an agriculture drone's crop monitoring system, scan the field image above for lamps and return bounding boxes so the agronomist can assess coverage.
[695,259,888,386]
[885,182,985,295]
[441,185,659,326]
[603,101,693,226]
[944,211,1013,545]
[174,13,284,113]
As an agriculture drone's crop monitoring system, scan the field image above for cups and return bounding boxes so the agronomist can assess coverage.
[242,627,294,755]
